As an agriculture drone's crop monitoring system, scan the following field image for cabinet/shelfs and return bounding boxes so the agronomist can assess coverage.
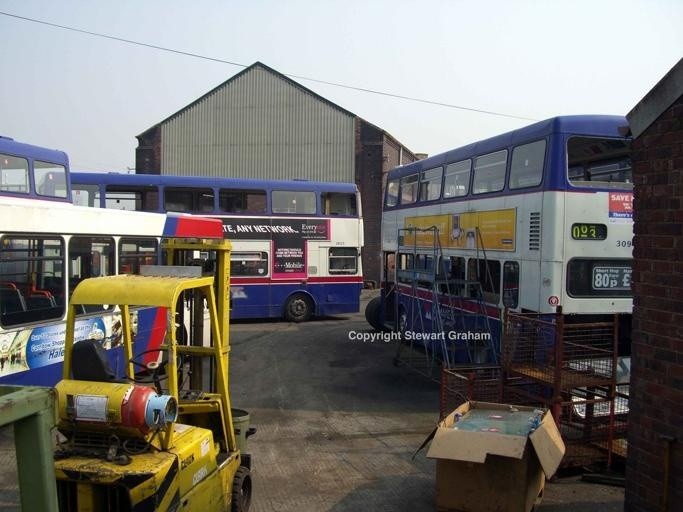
[502,304,619,469]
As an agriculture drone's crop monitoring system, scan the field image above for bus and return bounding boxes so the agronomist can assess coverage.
[364,114,634,392]
[0,136,74,204]
[0,196,224,389]
[37,173,362,320]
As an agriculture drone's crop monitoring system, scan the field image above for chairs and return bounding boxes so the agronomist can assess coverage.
[71,339,133,384]
[1,281,86,316]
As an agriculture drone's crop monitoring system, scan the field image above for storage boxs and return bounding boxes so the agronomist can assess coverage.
[413,400,567,512]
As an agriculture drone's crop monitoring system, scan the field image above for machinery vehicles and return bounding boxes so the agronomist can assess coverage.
[51,233,253,511]
